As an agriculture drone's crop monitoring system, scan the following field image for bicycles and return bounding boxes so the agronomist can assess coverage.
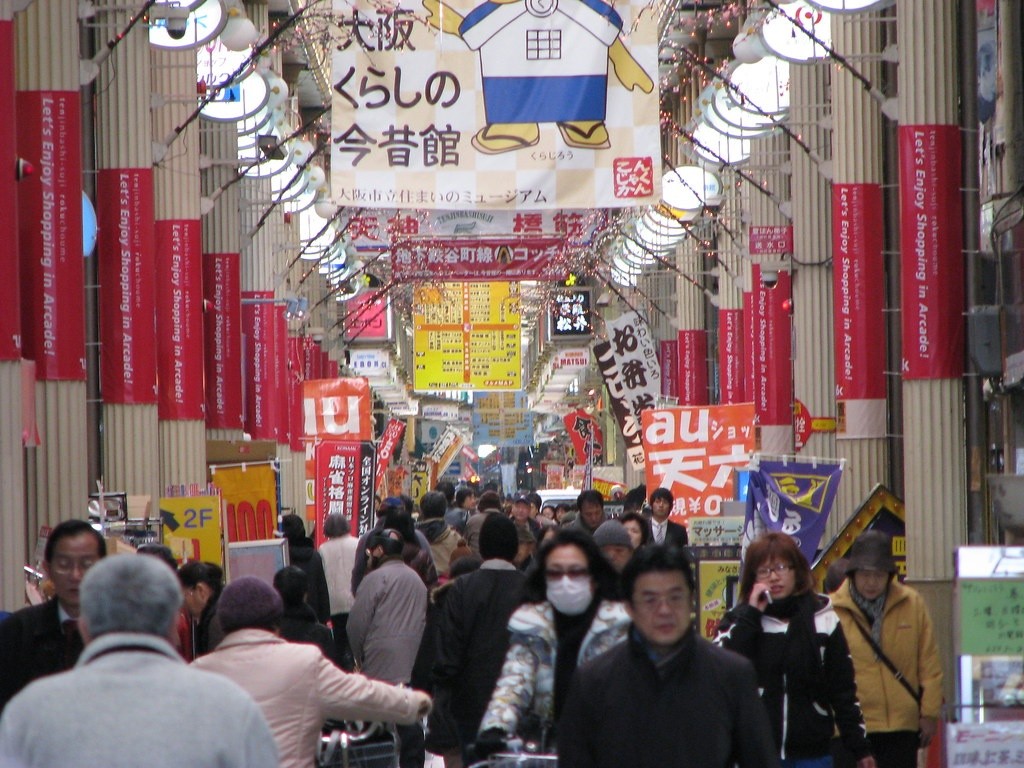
[469,735,559,768]
[317,711,431,768]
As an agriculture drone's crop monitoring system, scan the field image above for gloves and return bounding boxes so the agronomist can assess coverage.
[476,727,508,755]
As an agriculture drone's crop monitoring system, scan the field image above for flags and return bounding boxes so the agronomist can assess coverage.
[641,403,756,533]
[375,419,404,494]
[429,424,466,481]
[214,465,278,543]
[739,460,842,583]
[592,310,662,473]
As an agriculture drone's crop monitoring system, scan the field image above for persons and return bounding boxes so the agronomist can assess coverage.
[0,480,947,768]
[0,553,281,768]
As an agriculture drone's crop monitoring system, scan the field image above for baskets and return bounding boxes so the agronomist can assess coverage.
[317,732,399,768]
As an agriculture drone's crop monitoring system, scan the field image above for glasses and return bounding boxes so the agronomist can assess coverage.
[633,592,688,608]
[50,558,93,575]
[546,569,588,579]
[757,564,793,577]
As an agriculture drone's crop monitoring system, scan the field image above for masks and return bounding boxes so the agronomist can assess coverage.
[547,576,592,615]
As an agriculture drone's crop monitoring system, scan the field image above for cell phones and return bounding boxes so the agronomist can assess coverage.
[758,591,774,604]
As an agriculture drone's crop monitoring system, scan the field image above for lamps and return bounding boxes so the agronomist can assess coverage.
[730,2,775,62]
[242,295,309,322]
[201,6,365,277]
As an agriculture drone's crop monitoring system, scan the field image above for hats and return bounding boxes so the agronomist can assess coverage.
[478,513,519,555]
[217,577,281,633]
[593,520,632,550]
[368,530,404,555]
[514,495,530,506]
[842,532,894,574]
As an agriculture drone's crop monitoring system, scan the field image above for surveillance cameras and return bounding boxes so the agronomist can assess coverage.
[258,135,288,160]
[312,334,323,344]
[762,272,778,288]
[643,268,650,276]
[595,293,612,307]
[165,18,186,39]
[702,195,727,212]
[338,280,356,294]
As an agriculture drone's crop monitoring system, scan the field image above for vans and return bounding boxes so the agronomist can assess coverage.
[537,487,582,512]
